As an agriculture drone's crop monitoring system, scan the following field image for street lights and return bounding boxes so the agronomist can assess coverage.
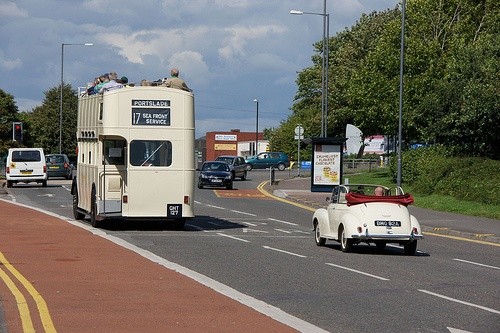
[252,98,259,156]
[288,10,330,139]
[59,42,94,155]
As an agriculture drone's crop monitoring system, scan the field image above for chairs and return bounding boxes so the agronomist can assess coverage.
[206,166,211,171]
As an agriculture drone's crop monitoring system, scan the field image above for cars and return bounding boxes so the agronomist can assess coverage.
[311,184,424,255]
[195,161,234,190]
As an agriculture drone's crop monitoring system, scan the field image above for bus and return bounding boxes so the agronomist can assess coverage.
[70,81,197,229]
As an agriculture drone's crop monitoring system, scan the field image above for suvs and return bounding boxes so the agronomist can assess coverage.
[215,155,249,180]
[46,154,74,179]
[245,151,290,172]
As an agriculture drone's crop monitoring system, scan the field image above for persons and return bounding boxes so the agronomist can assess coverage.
[373,186,385,197]
[99,71,123,93]
[166,68,193,91]
[81,73,149,97]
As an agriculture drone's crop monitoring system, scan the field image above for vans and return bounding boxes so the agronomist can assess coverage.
[5,148,49,187]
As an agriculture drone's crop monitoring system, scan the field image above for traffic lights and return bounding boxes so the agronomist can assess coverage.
[12,122,23,141]
